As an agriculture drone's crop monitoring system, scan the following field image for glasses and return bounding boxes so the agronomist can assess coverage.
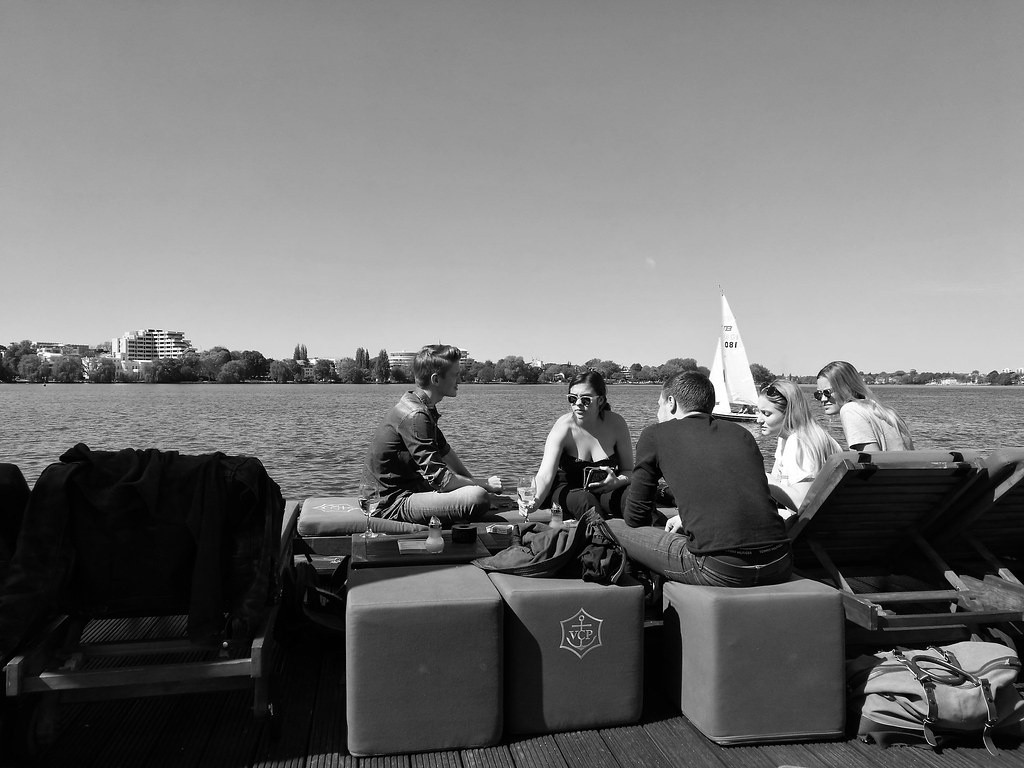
[760,381,788,404]
[567,394,599,406]
[814,389,835,400]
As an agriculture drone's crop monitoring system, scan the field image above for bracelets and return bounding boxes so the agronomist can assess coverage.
[660,485,669,498]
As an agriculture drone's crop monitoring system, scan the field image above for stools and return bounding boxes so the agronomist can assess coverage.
[661,575,844,746]
[486,567,644,741]
[342,564,502,758]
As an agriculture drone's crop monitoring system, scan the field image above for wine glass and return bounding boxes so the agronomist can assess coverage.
[517,476,536,523]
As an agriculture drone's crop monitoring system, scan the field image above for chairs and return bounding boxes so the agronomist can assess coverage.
[0,444,303,729]
[779,445,1024,650]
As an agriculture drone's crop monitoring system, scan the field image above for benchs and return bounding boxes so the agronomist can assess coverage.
[293,494,553,603]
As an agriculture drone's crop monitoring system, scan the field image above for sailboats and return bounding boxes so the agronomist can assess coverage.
[707,291,761,423]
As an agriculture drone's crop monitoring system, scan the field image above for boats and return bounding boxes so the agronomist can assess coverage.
[925,380,942,386]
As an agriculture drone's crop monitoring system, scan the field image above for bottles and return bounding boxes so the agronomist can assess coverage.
[425,515,444,554]
[549,501,565,530]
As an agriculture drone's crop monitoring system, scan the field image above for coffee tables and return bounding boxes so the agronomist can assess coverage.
[350,530,492,569]
[478,530,522,556]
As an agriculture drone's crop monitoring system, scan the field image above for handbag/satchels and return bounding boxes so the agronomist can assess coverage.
[848,640,1023,757]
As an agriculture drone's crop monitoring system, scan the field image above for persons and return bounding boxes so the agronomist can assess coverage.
[357,344,504,524]
[814,361,915,451]
[517,370,666,599]
[604,370,792,619]
[756,379,844,521]
